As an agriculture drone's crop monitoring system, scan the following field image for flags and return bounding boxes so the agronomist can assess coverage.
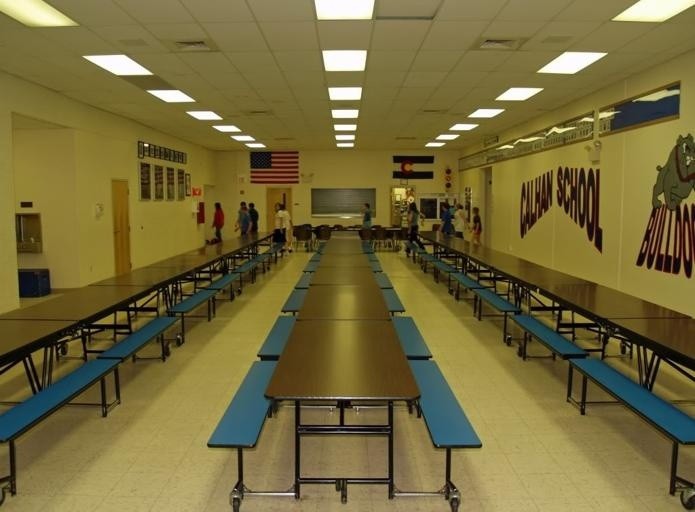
[249,151,298,183]
[393,155,435,180]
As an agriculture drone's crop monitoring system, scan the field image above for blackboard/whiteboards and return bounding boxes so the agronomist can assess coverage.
[310,188,376,218]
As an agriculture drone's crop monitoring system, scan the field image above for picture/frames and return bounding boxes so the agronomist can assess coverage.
[138,141,191,201]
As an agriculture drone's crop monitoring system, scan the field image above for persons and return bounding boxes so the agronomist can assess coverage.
[437,202,482,244]
[404,203,426,255]
[360,203,371,228]
[234,200,294,255]
[212,202,224,242]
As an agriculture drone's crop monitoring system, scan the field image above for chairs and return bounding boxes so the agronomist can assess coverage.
[292,224,407,252]
